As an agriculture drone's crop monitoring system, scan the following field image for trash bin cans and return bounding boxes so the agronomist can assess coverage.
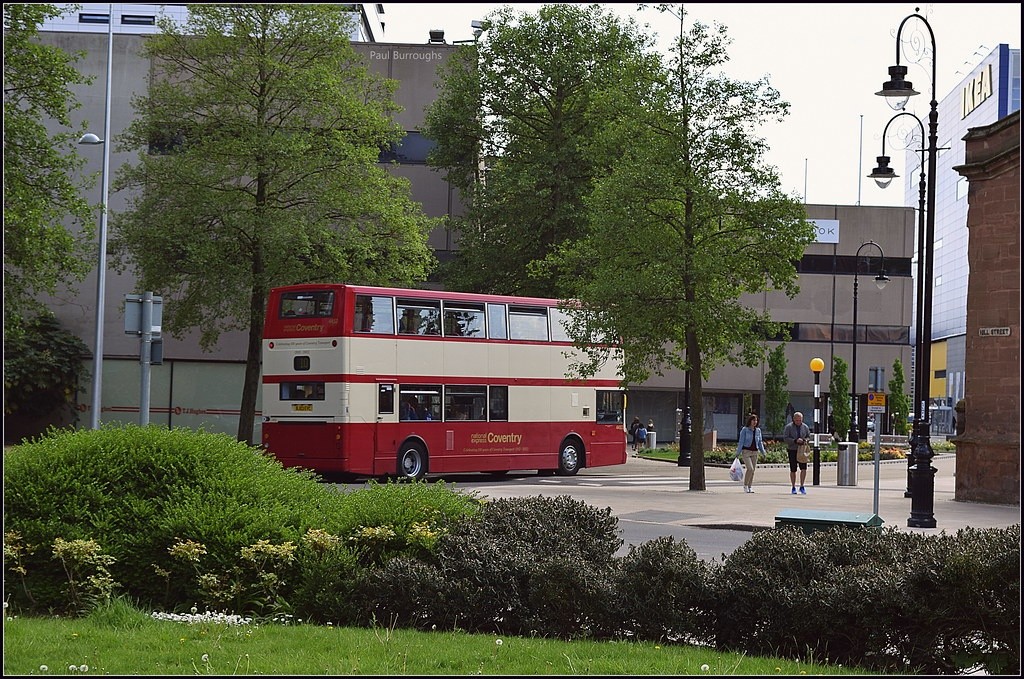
[647,432,656,451]
[837,441,857,486]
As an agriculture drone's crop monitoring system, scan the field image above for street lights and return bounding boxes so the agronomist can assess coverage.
[875,13,940,527]
[809,357,824,486]
[849,241,891,444]
[867,112,923,500]
[77,130,111,438]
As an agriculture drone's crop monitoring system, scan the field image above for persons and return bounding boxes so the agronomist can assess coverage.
[783,411,811,494]
[406,396,422,419]
[828,411,835,434]
[953,416,957,433]
[632,417,655,452]
[787,413,792,423]
[736,413,767,493]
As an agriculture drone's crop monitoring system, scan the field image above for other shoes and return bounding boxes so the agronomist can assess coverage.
[743,485,749,493]
[748,487,754,493]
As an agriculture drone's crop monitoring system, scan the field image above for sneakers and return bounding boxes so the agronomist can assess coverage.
[799,486,806,494]
[791,488,797,494]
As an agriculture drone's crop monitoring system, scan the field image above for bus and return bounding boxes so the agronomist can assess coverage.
[259,281,630,485]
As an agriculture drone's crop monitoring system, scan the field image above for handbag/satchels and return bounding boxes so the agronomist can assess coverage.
[797,441,810,463]
[729,457,743,481]
